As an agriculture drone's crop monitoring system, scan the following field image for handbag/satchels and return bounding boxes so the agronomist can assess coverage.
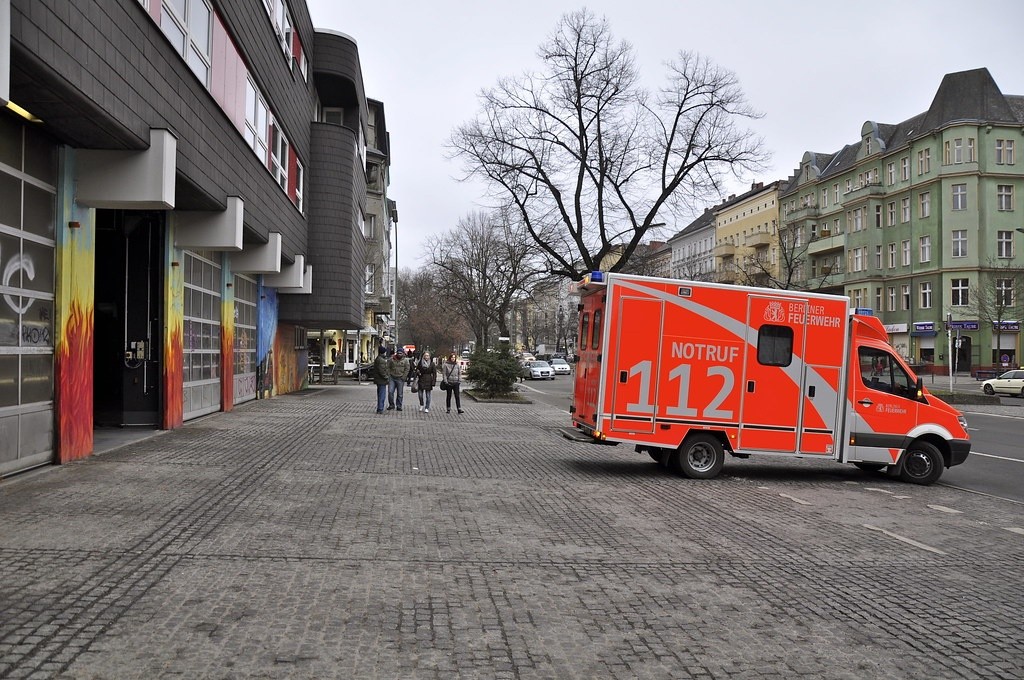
[412,376,419,392]
[440,380,446,391]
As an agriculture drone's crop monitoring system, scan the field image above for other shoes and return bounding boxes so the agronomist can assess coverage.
[419,405,424,411]
[446,410,450,413]
[397,406,402,411]
[458,409,464,414]
[377,409,383,413]
[387,405,395,410]
[425,408,428,413]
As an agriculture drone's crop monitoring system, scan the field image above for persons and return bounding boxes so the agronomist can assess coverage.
[387,348,410,411]
[871,355,884,376]
[443,353,464,414]
[373,345,390,415]
[417,351,437,413]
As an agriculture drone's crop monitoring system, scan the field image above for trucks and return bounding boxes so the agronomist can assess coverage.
[557,271,971,484]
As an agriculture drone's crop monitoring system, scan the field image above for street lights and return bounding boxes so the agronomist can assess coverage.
[391,209,397,354]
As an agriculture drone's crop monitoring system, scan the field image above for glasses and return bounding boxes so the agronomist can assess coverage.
[397,353,402,354]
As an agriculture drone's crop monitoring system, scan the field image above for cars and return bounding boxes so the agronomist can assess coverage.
[548,359,571,375]
[514,352,555,381]
[352,362,375,381]
[551,353,566,361]
[980,370,1024,397]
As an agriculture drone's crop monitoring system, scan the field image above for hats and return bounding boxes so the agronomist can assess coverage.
[397,348,403,352]
[378,346,385,354]
